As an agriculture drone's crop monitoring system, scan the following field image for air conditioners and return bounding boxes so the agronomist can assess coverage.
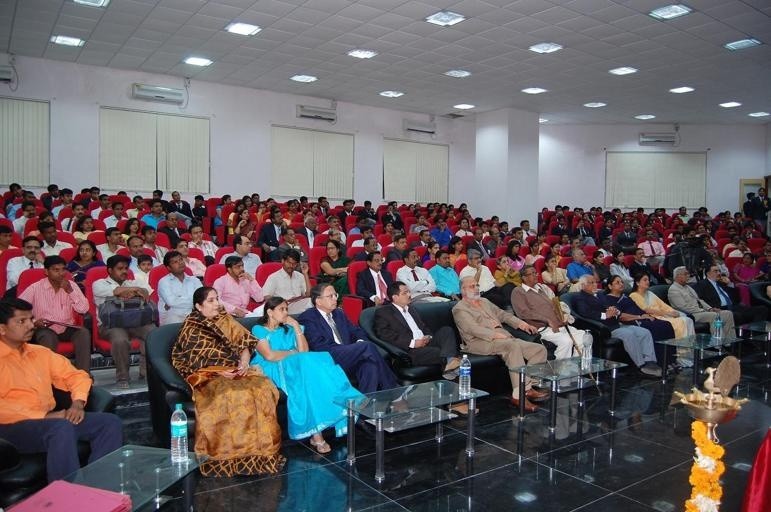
[296,103,339,121]
[638,131,681,145]
[130,80,188,107]
[0,65,14,83]
[404,116,438,135]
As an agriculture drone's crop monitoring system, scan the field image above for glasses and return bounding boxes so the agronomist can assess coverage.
[470,256,481,260]
[525,272,537,277]
[317,293,340,302]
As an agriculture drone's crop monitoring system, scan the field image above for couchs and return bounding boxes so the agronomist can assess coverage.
[559,281,770,370]
[145,291,583,446]
[0,362,119,512]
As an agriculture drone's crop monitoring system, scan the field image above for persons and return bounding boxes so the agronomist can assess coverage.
[0,296,124,482]
[172,286,289,480]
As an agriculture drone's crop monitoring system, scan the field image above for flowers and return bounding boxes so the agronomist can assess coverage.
[685,421,725,511]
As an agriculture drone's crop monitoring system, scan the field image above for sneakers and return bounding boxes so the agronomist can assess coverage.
[116,380,129,389]
[641,357,695,377]
[447,402,479,417]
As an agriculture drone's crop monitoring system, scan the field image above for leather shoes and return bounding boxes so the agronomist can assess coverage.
[526,388,551,402]
[510,395,538,412]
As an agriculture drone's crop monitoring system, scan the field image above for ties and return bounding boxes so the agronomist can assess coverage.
[649,240,656,256]
[715,281,734,306]
[326,313,343,345]
[28,261,35,268]
[377,273,391,304]
[411,269,419,281]
[580,227,586,237]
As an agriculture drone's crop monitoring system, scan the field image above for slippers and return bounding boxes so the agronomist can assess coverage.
[309,438,331,455]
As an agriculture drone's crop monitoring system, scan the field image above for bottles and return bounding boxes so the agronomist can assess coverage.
[581,362,593,374]
[459,354,472,396]
[167,402,188,461]
[713,313,724,339]
[582,329,594,361]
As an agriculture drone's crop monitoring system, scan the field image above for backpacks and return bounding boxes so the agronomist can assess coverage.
[97,293,158,330]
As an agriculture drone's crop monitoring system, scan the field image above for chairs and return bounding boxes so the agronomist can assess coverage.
[1,189,471,368]
[471,212,771,292]
